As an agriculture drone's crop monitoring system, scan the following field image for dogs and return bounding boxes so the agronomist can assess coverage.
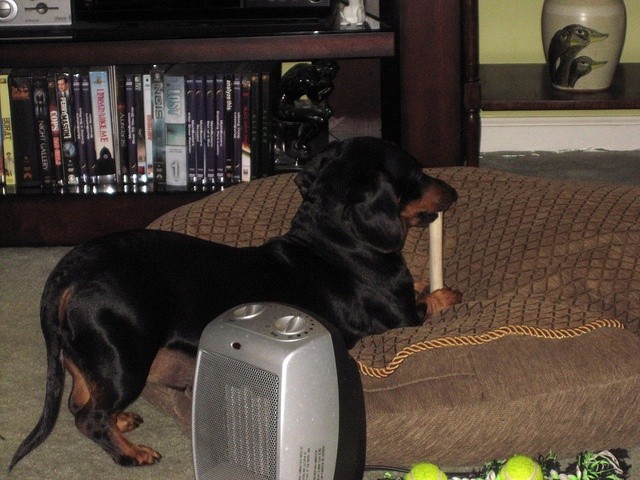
[5,138,463,475]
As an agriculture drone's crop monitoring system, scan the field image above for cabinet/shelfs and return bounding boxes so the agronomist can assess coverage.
[0,1,482,246]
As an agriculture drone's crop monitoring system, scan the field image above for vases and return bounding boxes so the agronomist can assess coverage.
[541,0,626,91]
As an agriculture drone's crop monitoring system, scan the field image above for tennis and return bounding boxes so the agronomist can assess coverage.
[404,462,449,480]
[500,456,544,480]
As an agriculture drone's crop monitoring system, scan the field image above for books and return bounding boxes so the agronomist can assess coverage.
[56,65,79,187]
[108,62,195,184]
[82,75,96,185]
[46,69,62,190]
[10,63,42,187]
[32,67,55,194]
[72,71,87,181]
[88,68,116,185]
[0,68,18,185]
[196,64,270,181]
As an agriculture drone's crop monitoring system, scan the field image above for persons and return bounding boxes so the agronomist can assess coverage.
[96,145,115,175]
[5,151,12,176]
[55,75,71,99]
